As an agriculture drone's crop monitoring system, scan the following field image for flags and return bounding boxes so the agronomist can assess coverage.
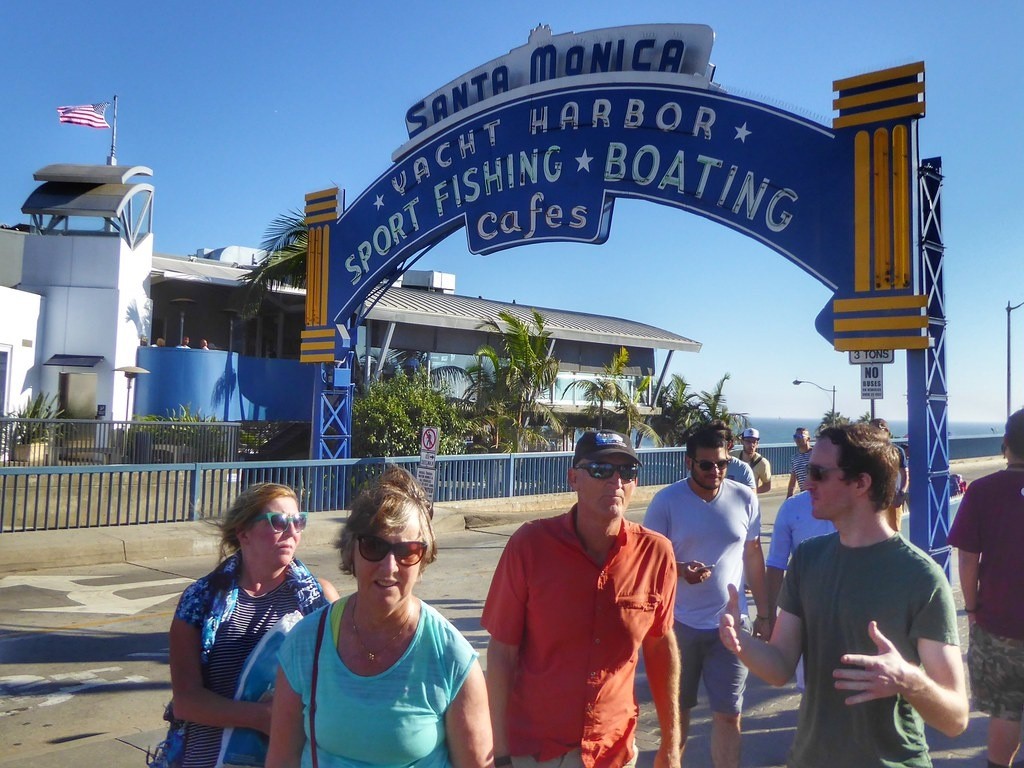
[57,101,110,129]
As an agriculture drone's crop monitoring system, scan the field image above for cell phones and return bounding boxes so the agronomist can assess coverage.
[696,565,715,573]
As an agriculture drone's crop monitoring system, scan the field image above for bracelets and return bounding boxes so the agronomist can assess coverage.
[965,608,976,613]
[756,615,768,620]
[899,491,904,496]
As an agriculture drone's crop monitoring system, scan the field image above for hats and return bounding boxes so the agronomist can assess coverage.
[742,428,760,441]
[575,430,642,467]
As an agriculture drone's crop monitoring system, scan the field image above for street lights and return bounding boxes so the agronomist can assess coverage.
[792,380,836,427]
[112,367,152,465]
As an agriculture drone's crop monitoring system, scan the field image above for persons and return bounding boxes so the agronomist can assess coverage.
[480,430,681,768]
[786,427,815,500]
[264,466,496,768]
[150,482,340,768]
[152,338,165,347]
[869,418,909,533]
[718,425,971,768]
[643,420,771,768]
[176,336,190,348]
[734,428,773,493]
[946,407,1024,768]
[764,491,839,693]
[200,339,209,350]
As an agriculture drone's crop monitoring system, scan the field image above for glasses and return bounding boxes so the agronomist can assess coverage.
[253,512,308,533]
[804,463,848,482]
[354,534,428,567]
[575,461,640,481]
[793,434,807,440]
[744,440,758,446]
[691,457,730,470]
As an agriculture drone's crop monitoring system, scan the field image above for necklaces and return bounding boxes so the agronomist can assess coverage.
[351,608,410,663]
